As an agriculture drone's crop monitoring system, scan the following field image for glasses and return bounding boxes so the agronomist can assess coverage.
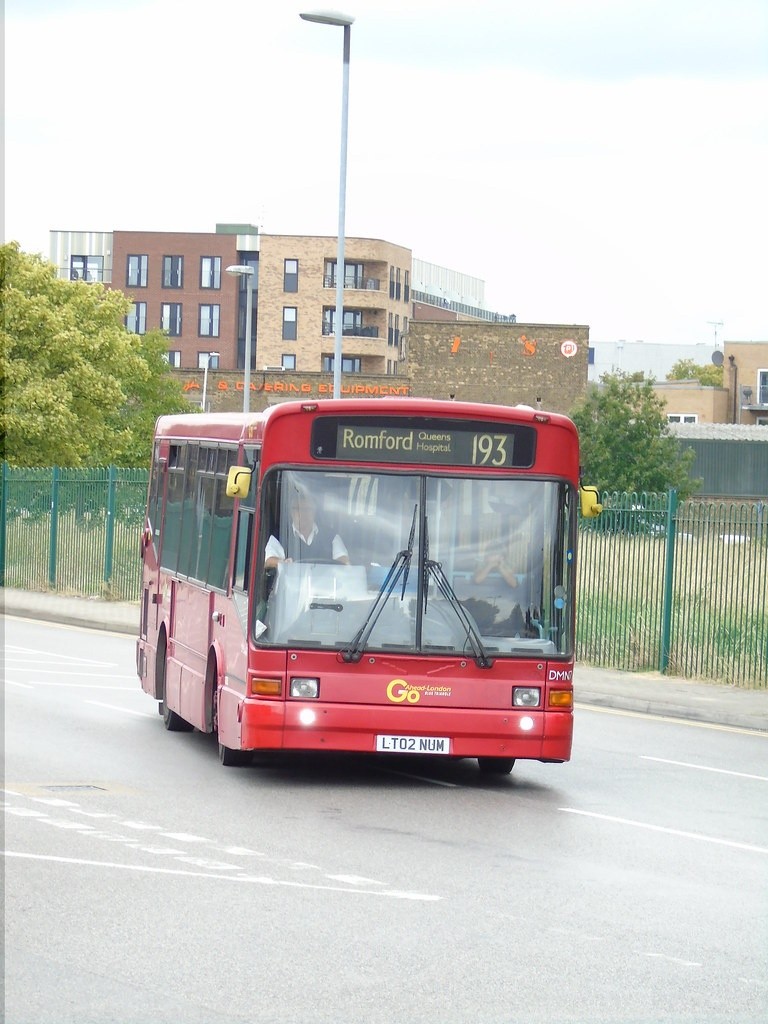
[292,506,314,512]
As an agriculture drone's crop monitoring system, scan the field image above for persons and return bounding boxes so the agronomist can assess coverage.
[474,540,519,589]
[264,490,351,601]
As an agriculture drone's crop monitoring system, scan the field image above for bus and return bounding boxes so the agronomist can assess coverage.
[134,395,601,774]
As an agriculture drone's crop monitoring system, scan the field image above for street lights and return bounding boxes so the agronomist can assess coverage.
[224,265,255,413]
[297,6,355,398]
[202,351,220,412]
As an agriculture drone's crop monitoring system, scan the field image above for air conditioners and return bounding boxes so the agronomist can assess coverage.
[263,366,285,371]
